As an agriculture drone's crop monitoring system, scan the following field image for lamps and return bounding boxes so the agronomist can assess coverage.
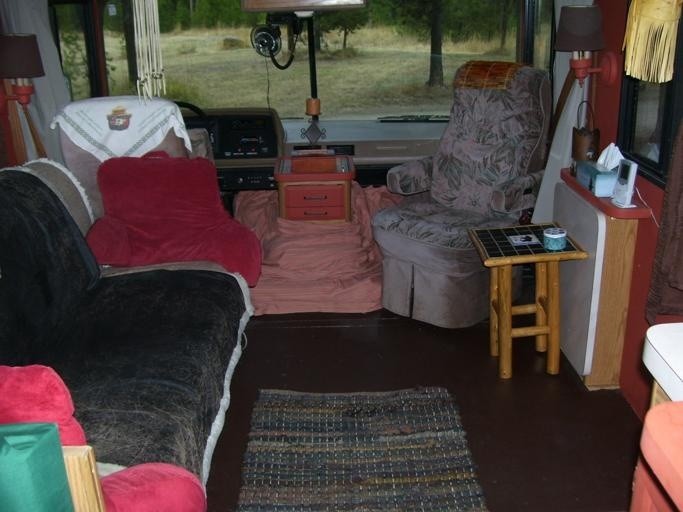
[551,5,606,91]
[0,32,46,111]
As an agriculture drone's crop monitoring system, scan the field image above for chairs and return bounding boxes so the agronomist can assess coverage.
[54,94,216,220]
[369,60,554,330]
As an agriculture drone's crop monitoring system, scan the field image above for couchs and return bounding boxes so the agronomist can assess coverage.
[0,157,257,500]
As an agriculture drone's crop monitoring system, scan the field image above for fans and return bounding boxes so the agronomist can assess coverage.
[251,24,285,58]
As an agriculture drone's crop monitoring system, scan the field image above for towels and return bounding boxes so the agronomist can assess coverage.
[644,118,682,326]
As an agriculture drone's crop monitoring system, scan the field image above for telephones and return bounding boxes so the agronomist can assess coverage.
[611,159,638,210]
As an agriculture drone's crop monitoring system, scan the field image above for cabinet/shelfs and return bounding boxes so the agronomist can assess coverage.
[273,154,357,224]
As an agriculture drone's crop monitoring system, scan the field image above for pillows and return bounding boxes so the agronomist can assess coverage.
[0,364,208,512]
[83,153,261,290]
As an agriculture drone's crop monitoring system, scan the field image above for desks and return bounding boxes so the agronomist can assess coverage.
[466,221,589,380]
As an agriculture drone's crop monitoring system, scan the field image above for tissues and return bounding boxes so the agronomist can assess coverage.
[577,143,626,198]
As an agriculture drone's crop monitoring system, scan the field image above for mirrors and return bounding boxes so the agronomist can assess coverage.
[615,0,683,192]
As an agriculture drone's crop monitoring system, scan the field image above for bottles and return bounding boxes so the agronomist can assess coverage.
[543,228,567,251]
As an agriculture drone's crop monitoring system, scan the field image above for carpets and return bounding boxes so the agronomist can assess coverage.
[235,383,490,512]
